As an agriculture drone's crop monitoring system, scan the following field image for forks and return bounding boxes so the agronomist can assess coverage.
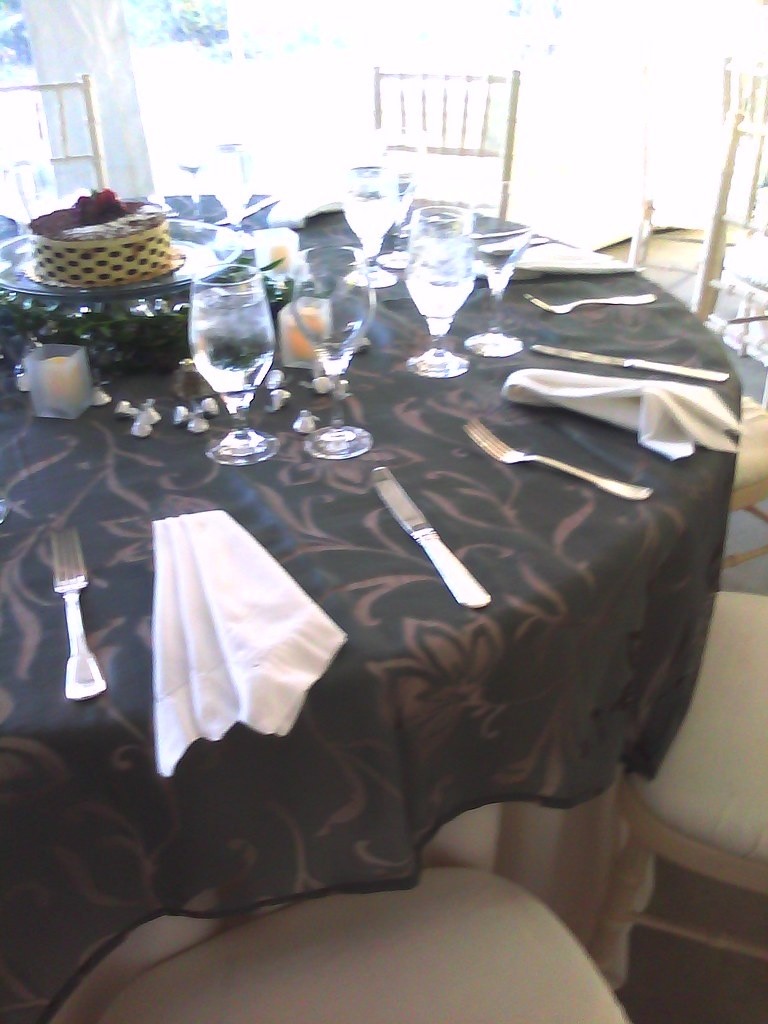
[523,292,658,314]
[50,526,107,702]
[463,416,656,502]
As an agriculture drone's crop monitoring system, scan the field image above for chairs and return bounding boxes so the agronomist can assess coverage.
[73,590,768,1024]
[685,112,768,567]
[373,61,520,225]
[0,73,115,220]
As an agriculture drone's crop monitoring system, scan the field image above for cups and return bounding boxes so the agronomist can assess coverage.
[253,227,299,290]
[276,296,331,369]
[24,344,96,420]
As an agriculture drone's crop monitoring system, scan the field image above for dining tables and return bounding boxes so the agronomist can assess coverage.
[0,188,768,1024]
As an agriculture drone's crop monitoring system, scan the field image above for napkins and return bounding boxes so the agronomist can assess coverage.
[151,510,350,778]
[498,366,746,461]
[265,195,368,229]
[512,242,646,277]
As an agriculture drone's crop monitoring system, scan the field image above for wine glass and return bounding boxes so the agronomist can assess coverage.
[290,243,376,460]
[188,263,280,466]
[339,129,427,289]
[404,205,476,379]
[463,174,532,357]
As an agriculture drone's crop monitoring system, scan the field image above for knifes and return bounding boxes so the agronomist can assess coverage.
[371,466,491,609]
[530,343,730,382]
